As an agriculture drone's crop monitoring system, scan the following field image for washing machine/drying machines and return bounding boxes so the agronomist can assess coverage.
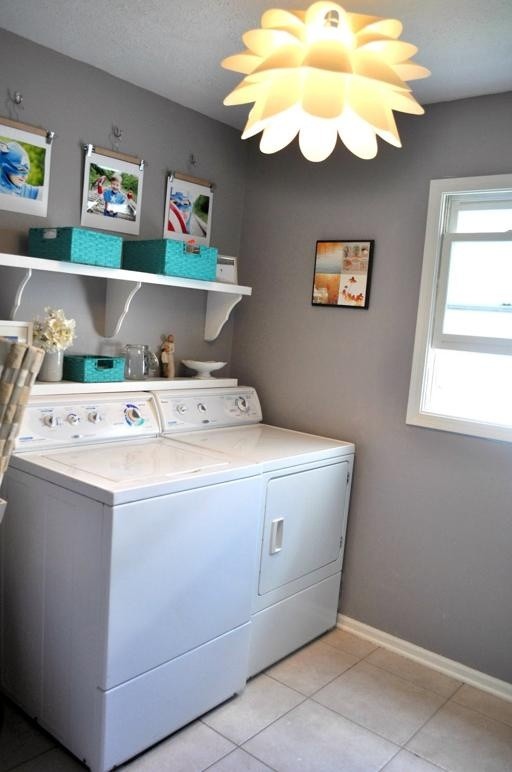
[8,393,265,772]
[156,384,357,678]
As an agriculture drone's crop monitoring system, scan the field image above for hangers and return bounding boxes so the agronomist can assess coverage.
[82,134,145,172]
[170,158,217,193]
[0,97,56,145]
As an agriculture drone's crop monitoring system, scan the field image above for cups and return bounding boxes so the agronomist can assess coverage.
[124,343,146,380]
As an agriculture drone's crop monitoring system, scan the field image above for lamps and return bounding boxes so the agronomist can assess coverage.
[218,0,432,162]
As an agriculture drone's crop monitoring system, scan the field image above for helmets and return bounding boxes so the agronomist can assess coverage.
[0,142,29,177]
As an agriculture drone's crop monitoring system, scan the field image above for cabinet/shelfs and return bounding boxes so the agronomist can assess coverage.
[0,252,253,395]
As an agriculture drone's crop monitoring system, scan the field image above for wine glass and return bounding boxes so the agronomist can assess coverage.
[181,358,228,380]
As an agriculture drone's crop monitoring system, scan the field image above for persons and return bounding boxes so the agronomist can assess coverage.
[171,187,193,235]
[0,142,44,201]
[159,334,175,379]
[97,174,135,217]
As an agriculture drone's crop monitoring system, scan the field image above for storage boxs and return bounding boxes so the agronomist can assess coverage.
[216,254,238,284]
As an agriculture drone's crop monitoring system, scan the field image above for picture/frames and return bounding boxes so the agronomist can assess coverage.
[309,236,375,311]
